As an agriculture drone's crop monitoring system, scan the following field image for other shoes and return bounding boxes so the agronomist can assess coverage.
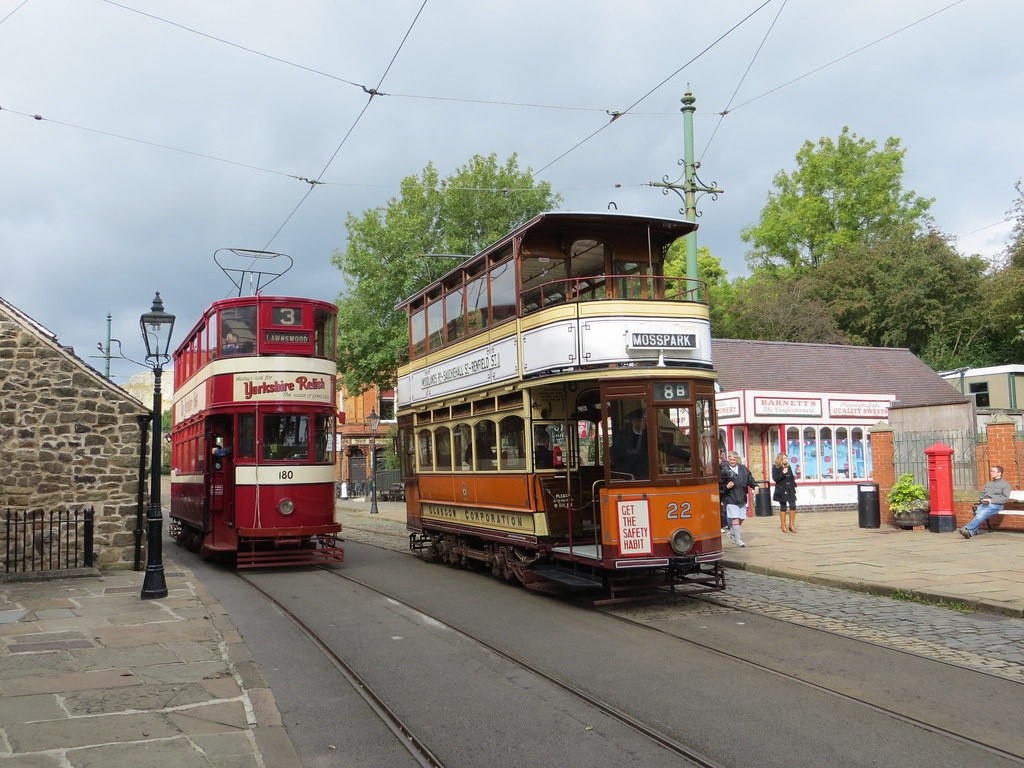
[720,528,725,533]
[960,527,971,539]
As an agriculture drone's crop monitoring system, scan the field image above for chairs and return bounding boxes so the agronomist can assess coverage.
[411,301,610,494]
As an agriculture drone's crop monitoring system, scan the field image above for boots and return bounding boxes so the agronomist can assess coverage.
[731,523,745,547]
[780,512,788,532]
[728,529,735,544]
[789,510,797,533]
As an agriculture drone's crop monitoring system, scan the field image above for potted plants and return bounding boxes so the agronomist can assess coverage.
[887,473,930,527]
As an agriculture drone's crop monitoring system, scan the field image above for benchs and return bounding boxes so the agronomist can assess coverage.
[972,489,1024,534]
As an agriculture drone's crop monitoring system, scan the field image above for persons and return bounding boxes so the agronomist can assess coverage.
[211,426,233,528]
[957,466,1011,540]
[772,452,798,533]
[720,451,759,547]
[465,430,505,471]
[719,450,730,532]
[603,408,692,480]
[222,333,242,355]
[523,431,565,470]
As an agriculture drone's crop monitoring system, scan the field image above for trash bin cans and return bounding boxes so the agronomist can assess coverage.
[755,481,771,517]
[857,484,881,529]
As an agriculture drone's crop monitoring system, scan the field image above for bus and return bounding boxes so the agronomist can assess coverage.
[168,246,346,574]
[391,199,727,605]
